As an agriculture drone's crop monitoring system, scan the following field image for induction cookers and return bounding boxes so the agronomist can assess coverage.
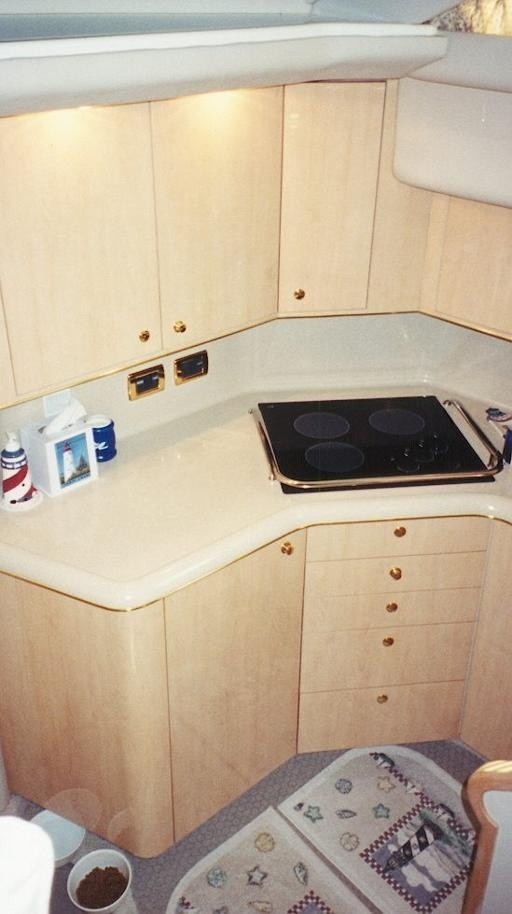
[256,396,494,494]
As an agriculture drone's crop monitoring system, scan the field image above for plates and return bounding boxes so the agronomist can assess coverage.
[30,806,85,866]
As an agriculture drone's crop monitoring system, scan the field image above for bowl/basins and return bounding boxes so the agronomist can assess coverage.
[66,848,134,913]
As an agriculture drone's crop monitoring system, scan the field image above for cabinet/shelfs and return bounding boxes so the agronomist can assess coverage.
[283,80,385,314]
[298,518,490,747]
[0,82,284,396]
[364,75,511,342]
[2,514,307,858]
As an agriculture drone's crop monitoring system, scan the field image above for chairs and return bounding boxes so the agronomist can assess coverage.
[460,758,512,914]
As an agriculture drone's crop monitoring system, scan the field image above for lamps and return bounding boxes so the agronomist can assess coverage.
[419,0,512,38]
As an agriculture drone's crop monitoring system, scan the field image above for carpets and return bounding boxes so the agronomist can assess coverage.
[165,804,373,914]
[277,745,477,914]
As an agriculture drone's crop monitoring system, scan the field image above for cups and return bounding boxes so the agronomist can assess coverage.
[88,415,117,463]
